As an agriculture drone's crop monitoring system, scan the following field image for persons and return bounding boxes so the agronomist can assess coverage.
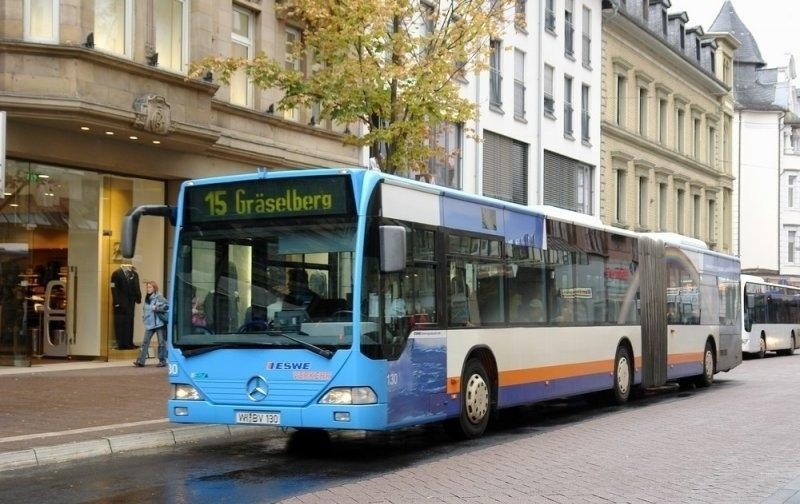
[366,274,406,323]
[227,258,241,333]
[133,281,168,367]
[283,267,319,303]
[109,256,141,351]
[190,297,208,324]
[438,257,631,324]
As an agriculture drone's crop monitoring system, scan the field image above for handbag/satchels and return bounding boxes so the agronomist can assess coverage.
[155,296,169,323]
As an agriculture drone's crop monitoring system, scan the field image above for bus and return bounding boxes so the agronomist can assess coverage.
[742,274,800,359]
[120,167,742,441]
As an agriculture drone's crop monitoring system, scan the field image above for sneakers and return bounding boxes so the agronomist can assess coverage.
[156,362,166,367]
[133,361,144,367]
